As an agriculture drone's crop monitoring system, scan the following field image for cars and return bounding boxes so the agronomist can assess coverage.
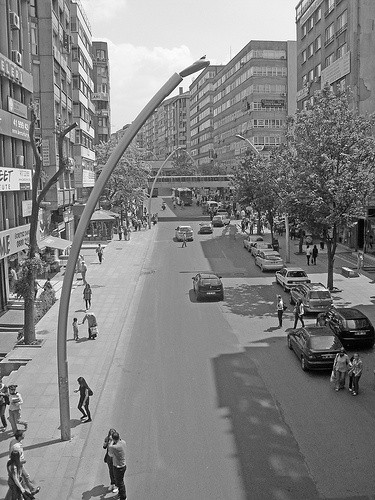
[192,273,224,300]
[175,225,194,241]
[274,267,312,292]
[287,325,347,372]
[198,222,214,234]
[210,210,231,227]
[242,235,284,272]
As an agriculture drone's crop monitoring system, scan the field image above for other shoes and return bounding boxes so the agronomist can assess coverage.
[81,415,92,424]
[32,485,40,495]
[335,386,359,396]
[108,485,128,500]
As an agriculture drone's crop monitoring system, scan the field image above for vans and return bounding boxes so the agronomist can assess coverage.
[204,201,218,212]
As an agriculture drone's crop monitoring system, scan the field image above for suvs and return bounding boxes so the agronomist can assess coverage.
[316,308,375,350]
[289,281,334,316]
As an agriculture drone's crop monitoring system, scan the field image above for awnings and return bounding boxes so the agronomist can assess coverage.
[41,235,72,251]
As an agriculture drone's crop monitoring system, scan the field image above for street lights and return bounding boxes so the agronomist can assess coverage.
[56,57,212,441]
[235,134,291,263]
[148,146,181,229]
[183,148,203,181]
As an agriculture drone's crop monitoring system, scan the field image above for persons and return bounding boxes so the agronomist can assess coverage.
[273,222,285,236]
[72,318,82,340]
[180,199,186,210]
[290,225,296,240]
[8,429,39,494]
[312,245,318,265]
[80,262,88,285]
[294,298,305,328]
[103,428,121,493]
[82,310,97,340]
[119,225,132,241]
[162,201,167,211]
[356,252,363,276]
[9,267,17,292]
[133,207,159,231]
[82,284,92,310]
[34,277,52,299]
[277,295,284,328]
[172,199,177,210]
[5,449,24,500]
[195,187,240,220]
[181,232,187,248]
[73,377,92,423]
[306,245,311,265]
[0,379,10,432]
[333,347,363,396]
[96,243,103,264]
[8,385,28,437]
[241,205,268,235]
[107,431,127,500]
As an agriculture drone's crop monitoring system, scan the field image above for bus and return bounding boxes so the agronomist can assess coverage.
[170,188,193,205]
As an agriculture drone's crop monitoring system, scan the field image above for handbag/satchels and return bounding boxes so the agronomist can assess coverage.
[283,303,288,312]
[329,371,336,383]
[90,326,98,335]
[311,257,314,264]
